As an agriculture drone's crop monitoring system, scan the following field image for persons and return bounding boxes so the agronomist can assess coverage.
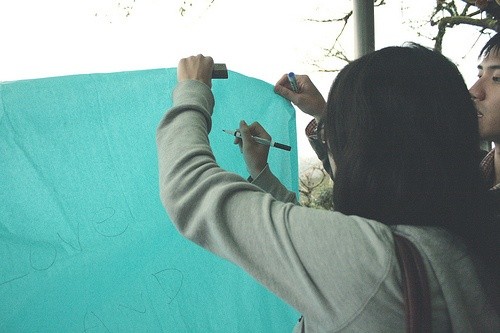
[274,30,500,210]
[153,42,499,333]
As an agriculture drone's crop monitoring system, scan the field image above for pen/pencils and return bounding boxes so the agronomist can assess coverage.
[289,72,300,93]
[222,129,291,152]
